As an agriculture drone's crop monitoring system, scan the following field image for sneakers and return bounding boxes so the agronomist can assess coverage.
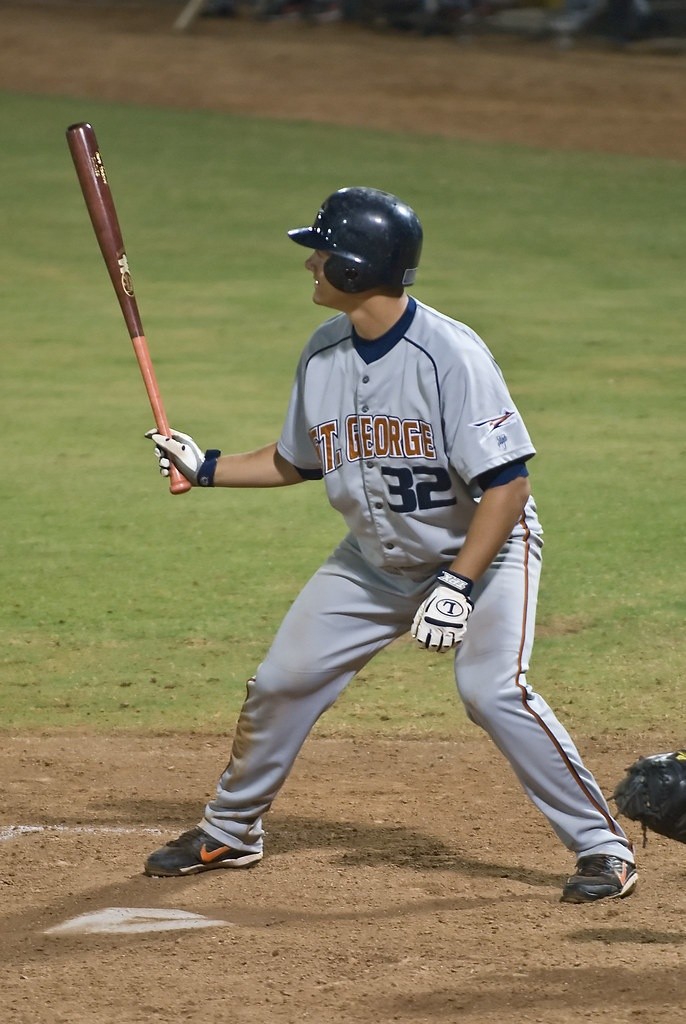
[562,851,640,903]
[145,826,264,876]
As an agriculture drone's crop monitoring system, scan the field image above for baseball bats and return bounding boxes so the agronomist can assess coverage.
[66,118,196,498]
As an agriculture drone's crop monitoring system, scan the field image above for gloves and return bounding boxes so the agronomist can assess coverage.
[410,567,476,653]
[143,428,220,487]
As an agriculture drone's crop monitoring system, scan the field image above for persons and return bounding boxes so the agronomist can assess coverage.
[144,188,641,904]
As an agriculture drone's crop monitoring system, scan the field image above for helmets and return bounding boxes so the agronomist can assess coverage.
[286,185,424,294]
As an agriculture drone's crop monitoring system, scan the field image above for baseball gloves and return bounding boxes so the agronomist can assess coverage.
[611,749,686,844]
